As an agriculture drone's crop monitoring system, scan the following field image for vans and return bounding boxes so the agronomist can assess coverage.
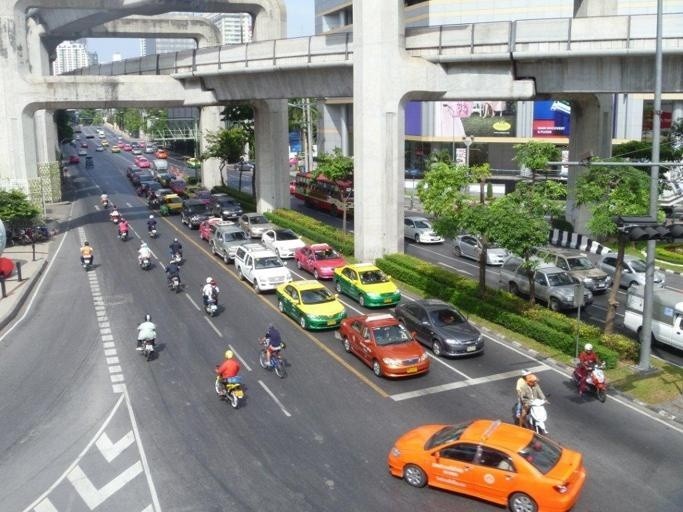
[624,284,683,353]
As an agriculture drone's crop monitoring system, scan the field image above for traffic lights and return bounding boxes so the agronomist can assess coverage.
[614,215,683,245]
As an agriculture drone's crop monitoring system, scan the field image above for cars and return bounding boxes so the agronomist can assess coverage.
[209,192,242,219]
[99,131,106,138]
[596,253,666,289]
[333,263,401,308]
[508,268,593,312]
[127,165,143,181]
[195,191,211,205]
[155,188,172,198]
[140,142,145,147]
[155,149,167,158]
[187,158,199,168]
[137,175,153,186]
[118,134,122,139]
[164,194,183,208]
[340,312,431,378]
[291,180,296,195]
[78,149,87,156]
[145,145,153,153]
[135,155,150,168]
[181,199,216,229]
[260,228,306,259]
[208,224,251,263]
[234,161,254,171]
[102,140,108,146]
[124,144,132,151]
[132,148,141,154]
[131,142,138,148]
[239,212,278,238]
[142,182,162,198]
[294,244,348,280]
[403,216,445,244]
[80,142,87,148]
[111,146,120,153]
[200,218,234,241]
[97,127,101,132]
[71,154,79,163]
[499,256,538,286]
[395,299,485,358]
[96,144,104,151]
[451,234,511,265]
[235,244,293,294]
[388,420,587,511]
[149,159,169,177]
[276,279,347,331]
[547,252,611,293]
[118,141,124,147]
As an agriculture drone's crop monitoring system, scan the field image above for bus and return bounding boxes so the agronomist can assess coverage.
[296,171,354,219]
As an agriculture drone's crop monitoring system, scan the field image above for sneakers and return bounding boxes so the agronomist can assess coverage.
[135,346,143,351]
[263,361,271,367]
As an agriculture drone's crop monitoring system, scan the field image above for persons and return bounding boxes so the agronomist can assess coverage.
[111,206,119,214]
[147,215,156,231]
[165,259,180,284]
[516,369,546,427]
[100,191,108,203]
[117,218,128,238]
[135,314,156,350]
[202,277,219,307]
[137,243,151,264]
[577,343,600,398]
[169,237,182,259]
[80,242,94,264]
[216,350,241,395]
[263,324,282,366]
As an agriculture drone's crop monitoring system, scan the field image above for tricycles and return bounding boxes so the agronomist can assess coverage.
[85,156,93,168]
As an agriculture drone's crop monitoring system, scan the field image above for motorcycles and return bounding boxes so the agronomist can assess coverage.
[147,223,157,238]
[167,275,180,294]
[574,362,606,402]
[513,393,550,434]
[215,364,244,409]
[169,250,183,264]
[118,224,128,240]
[136,323,156,361]
[136,247,152,269]
[80,254,93,269]
[102,200,109,207]
[112,216,119,224]
[201,284,218,317]
[257,337,287,379]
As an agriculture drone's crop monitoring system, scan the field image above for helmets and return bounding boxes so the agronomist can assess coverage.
[144,312,152,321]
[210,280,216,285]
[206,276,213,284]
[84,241,89,246]
[173,237,178,242]
[149,215,154,219]
[525,373,538,385]
[520,367,531,377]
[224,350,233,360]
[584,343,593,352]
